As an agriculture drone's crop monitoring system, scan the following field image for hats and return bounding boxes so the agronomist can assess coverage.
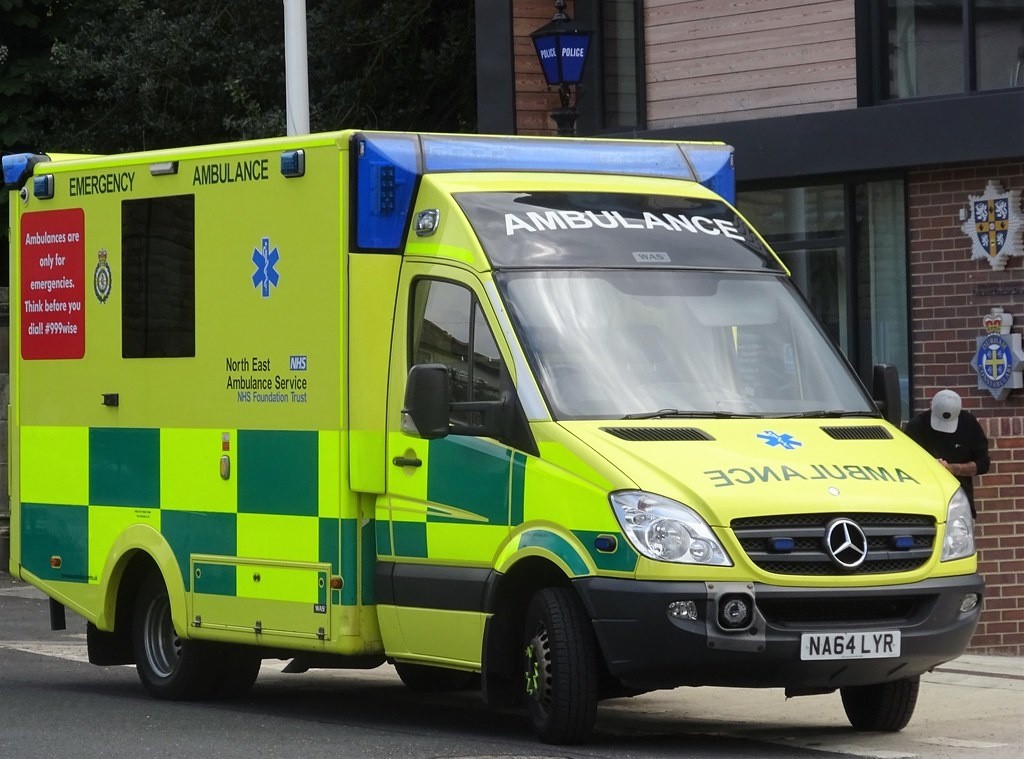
[930,389,961,433]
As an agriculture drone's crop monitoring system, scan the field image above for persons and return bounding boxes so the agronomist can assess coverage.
[905,390,991,532]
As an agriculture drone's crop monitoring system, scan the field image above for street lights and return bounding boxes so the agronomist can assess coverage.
[530,1,596,138]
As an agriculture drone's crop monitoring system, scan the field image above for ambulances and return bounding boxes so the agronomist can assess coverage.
[1,128,988,747]
